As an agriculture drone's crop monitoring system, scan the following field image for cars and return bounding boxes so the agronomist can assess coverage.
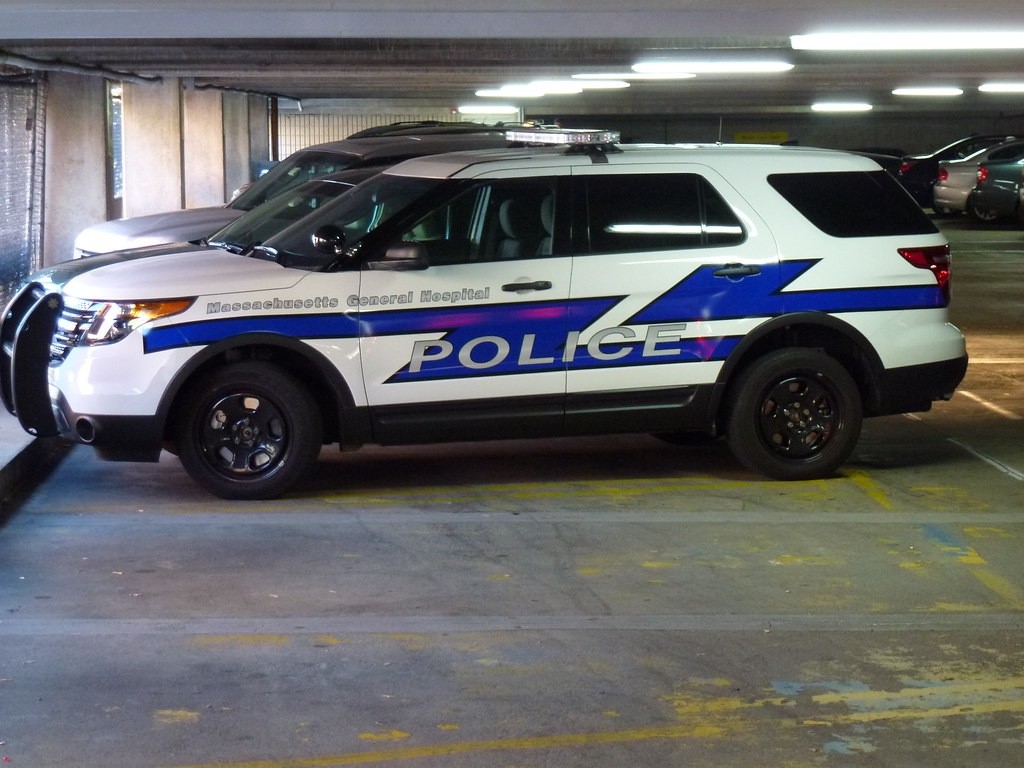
[837,133,1024,224]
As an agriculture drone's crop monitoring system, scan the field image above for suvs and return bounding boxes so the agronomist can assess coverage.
[74,120,902,262]
[0,127,968,500]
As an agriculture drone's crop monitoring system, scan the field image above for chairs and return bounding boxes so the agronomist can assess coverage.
[345,196,589,259]
